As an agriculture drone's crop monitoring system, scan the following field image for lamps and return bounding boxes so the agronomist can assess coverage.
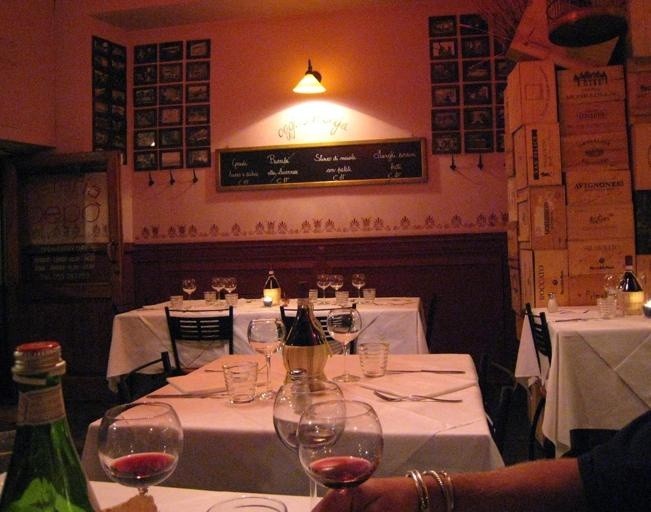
[290,59,327,95]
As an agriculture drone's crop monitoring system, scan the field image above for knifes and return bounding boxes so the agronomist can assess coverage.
[388,367,468,375]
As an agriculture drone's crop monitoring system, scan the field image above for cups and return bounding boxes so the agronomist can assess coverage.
[357,340,391,380]
[170,295,184,310]
[219,358,259,403]
[204,494,286,512]
[596,297,618,320]
[363,288,376,303]
[307,288,318,303]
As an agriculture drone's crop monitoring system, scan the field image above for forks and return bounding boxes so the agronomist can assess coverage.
[370,389,462,405]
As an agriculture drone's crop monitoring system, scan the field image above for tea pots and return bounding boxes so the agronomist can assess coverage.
[277,300,330,379]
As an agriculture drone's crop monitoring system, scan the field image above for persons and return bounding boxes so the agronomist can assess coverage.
[311,409,651,511]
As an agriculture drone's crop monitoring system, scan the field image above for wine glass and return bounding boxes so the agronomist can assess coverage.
[325,305,364,384]
[603,272,620,299]
[315,272,366,306]
[182,276,238,309]
[245,319,286,402]
[270,372,385,512]
[98,399,187,496]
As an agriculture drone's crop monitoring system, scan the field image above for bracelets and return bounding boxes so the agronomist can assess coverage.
[405,468,456,511]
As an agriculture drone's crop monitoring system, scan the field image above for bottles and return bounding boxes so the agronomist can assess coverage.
[262,270,281,305]
[617,254,643,317]
[546,291,559,313]
[0,341,103,512]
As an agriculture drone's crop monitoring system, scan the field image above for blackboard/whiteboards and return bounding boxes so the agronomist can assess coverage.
[214,136,428,191]
